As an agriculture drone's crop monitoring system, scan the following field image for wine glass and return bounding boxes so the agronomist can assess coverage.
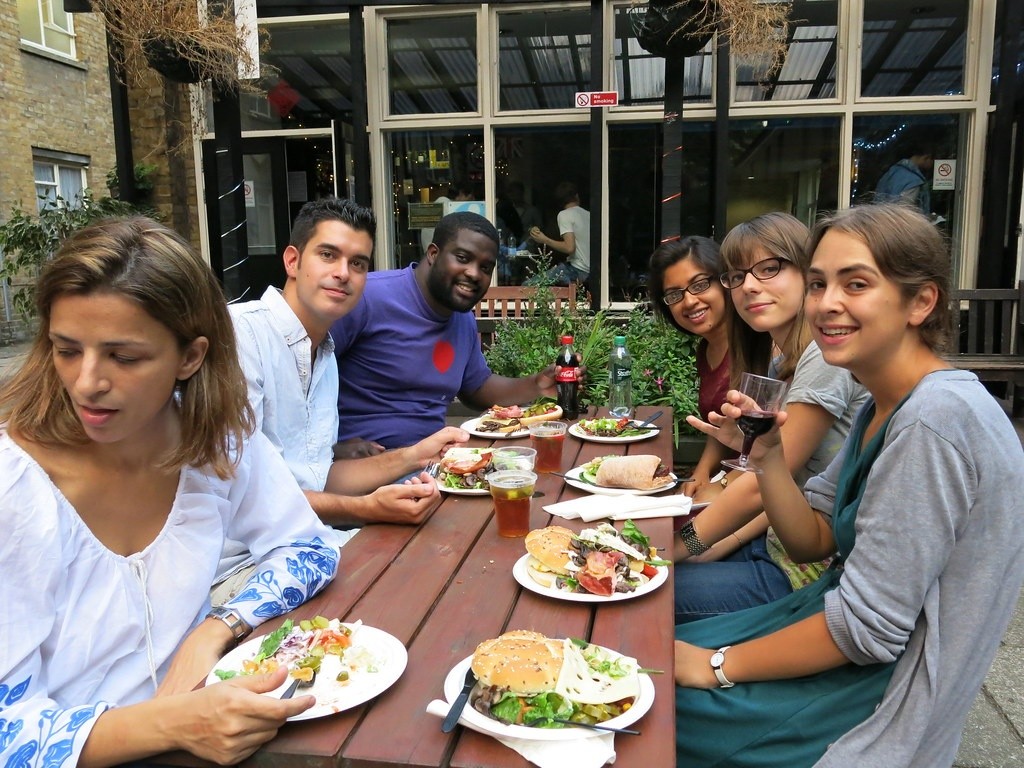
[720,372,788,474]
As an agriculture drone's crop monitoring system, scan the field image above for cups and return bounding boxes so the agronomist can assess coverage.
[528,420,567,473]
[491,446,538,470]
[488,470,539,537]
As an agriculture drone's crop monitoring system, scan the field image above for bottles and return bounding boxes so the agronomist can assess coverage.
[498,229,504,256]
[610,336,633,417]
[555,336,580,421]
[508,233,516,260]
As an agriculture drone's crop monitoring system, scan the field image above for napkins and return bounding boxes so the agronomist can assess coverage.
[425,700,617,768]
[542,494,693,523]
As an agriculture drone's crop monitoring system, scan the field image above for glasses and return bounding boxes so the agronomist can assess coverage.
[719,257,795,290]
[652,273,718,306]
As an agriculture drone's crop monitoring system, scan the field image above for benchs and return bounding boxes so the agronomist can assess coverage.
[475,284,576,350]
[938,280,1024,419]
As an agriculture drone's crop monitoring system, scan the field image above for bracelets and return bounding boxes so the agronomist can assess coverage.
[680,517,710,555]
[733,533,742,547]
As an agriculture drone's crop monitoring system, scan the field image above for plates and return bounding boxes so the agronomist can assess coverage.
[563,466,677,496]
[516,254,541,257]
[460,417,529,439]
[429,463,491,496]
[205,623,408,721]
[568,419,660,443]
[512,553,669,601]
[444,638,656,740]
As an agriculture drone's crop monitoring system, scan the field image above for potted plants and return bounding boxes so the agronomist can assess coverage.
[626,0,810,92]
[89,0,282,104]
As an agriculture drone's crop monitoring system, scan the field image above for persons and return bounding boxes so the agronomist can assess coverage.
[420,177,591,289]
[0,215,341,768]
[214,198,471,526]
[331,211,586,486]
[649,134,952,624]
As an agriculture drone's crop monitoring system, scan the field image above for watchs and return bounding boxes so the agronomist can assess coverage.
[720,476,728,489]
[709,646,734,688]
[205,606,247,648]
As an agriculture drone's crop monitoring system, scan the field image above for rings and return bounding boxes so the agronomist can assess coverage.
[678,491,685,495]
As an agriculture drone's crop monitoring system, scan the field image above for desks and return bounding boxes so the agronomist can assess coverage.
[186,405,675,768]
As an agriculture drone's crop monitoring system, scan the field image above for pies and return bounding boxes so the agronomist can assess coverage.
[595,455,673,490]
[480,405,563,432]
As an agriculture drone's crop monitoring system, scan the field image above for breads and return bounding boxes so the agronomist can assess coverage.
[524,525,580,576]
[470,630,562,693]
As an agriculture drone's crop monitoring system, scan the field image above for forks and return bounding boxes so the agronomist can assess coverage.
[505,429,519,438]
[527,717,641,735]
[414,461,438,502]
[625,421,662,431]
[280,671,316,699]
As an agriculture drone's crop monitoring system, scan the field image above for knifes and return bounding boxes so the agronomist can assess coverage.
[639,411,664,427]
[440,667,479,733]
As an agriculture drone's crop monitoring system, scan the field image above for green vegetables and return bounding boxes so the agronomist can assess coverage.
[620,519,673,566]
[578,417,650,436]
[215,618,295,681]
[445,449,485,489]
[590,463,602,475]
[491,660,613,728]
